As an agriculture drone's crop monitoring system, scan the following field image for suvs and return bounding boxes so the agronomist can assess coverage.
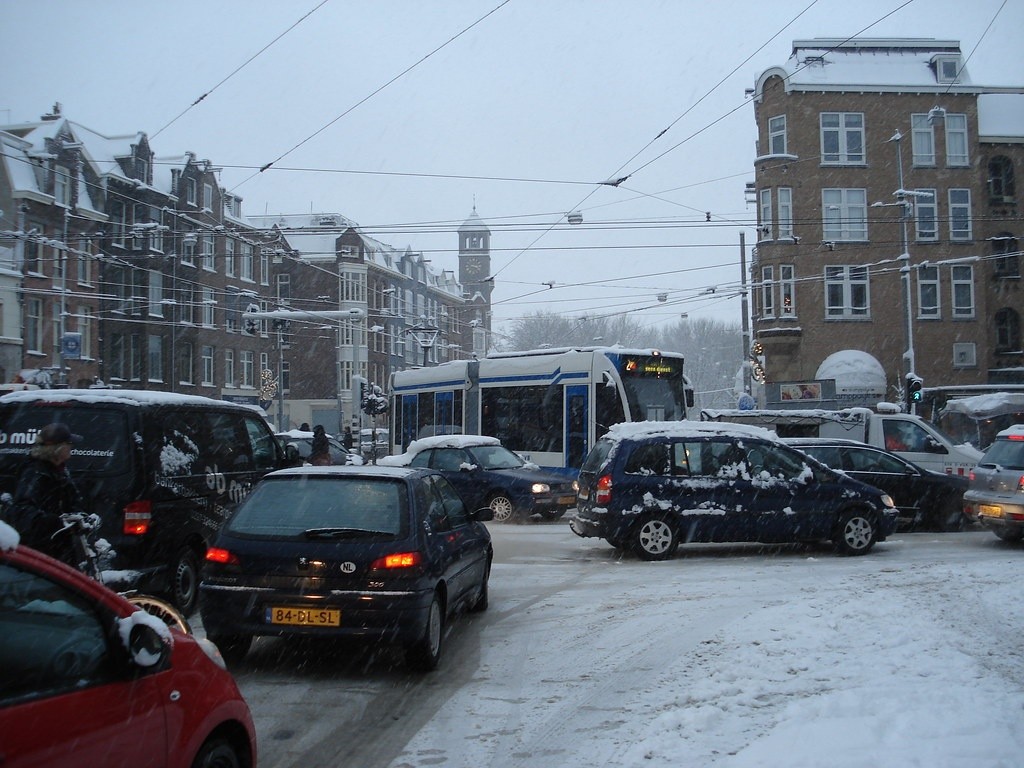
[963,423,1024,541]
[0,389,302,621]
[569,418,899,562]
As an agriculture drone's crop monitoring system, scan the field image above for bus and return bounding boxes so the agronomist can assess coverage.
[388,346,695,470]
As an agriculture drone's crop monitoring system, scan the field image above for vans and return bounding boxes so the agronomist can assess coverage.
[701,402,986,481]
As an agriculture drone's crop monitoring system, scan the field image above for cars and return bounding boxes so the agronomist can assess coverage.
[0,539,259,768]
[247,430,376,465]
[775,437,981,533]
[376,434,580,523]
[198,464,494,674]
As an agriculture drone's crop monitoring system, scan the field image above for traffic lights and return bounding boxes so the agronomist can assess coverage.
[909,379,923,402]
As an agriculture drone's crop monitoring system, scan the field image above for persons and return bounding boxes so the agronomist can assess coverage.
[885,427,912,451]
[302,424,330,466]
[0,503,88,608]
[13,426,82,571]
[342,426,352,450]
[299,423,311,432]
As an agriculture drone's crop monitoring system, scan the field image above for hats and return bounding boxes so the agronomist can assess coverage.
[39,423,84,445]
